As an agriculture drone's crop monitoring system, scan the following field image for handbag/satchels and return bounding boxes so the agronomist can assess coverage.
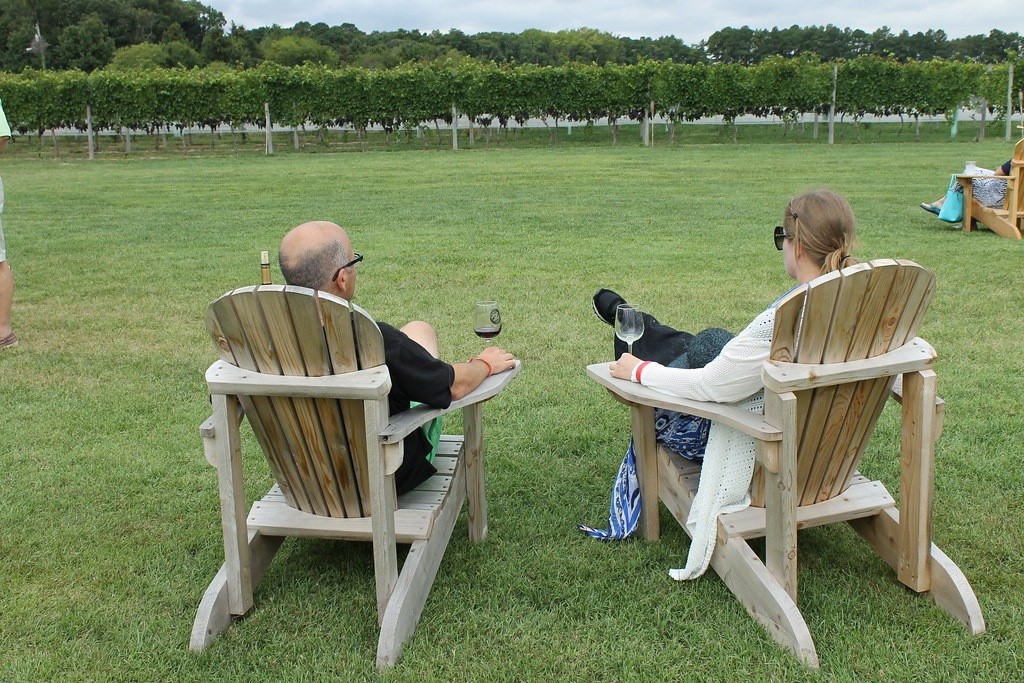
[938,174,963,223]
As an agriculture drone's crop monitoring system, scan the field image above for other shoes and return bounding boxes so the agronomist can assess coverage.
[0,330,18,349]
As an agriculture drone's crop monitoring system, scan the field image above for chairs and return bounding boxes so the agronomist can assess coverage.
[191,285,520,674]
[956,138,1024,240]
[585,257,986,670]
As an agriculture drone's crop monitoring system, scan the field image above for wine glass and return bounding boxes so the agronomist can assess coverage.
[473,300,502,348]
[615,304,643,354]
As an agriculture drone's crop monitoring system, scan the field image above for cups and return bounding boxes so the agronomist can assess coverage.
[966,161,975,174]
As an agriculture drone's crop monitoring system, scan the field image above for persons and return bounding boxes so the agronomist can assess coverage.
[592,189,858,505]
[919,157,1011,231]
[0,95,16,346]
[277,221,518,499]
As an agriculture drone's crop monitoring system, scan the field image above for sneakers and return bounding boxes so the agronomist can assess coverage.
[592,288,627,326]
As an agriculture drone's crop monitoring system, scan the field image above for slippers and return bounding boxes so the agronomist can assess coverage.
[919,202,941,215]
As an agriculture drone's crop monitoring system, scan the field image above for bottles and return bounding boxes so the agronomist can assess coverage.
[260,250,272,285]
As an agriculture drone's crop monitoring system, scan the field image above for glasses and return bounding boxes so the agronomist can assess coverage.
[774,227,787,250]
[332,252,363,282]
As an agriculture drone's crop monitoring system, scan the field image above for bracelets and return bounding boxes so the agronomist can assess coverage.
[469,357,492,379]
[636,361,651,383]
